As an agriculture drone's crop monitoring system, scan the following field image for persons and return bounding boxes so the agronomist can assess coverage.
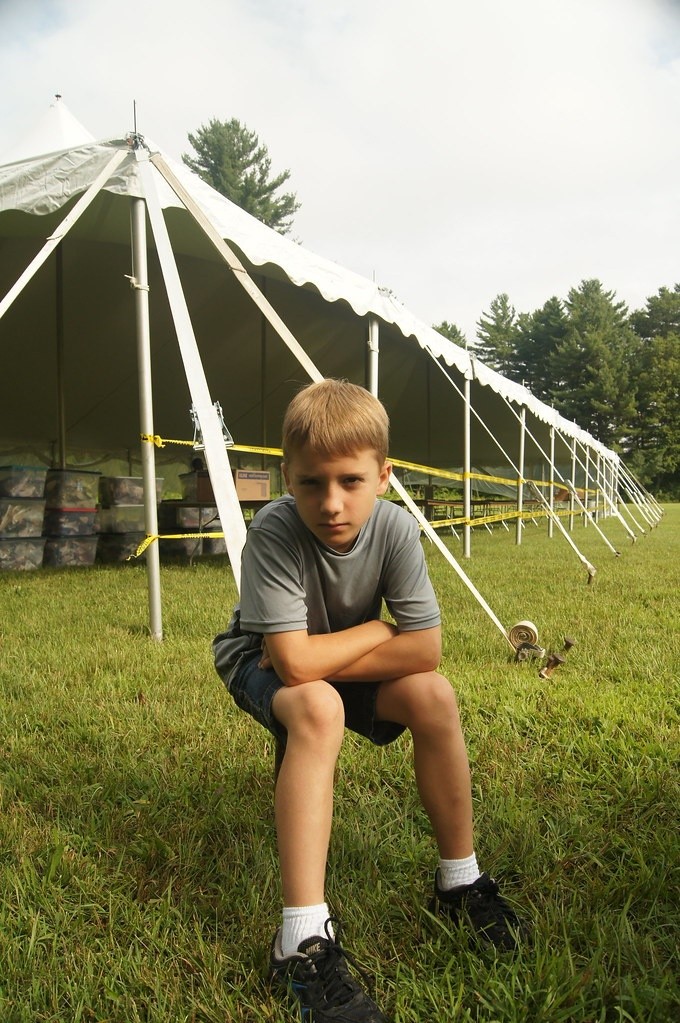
[211,380,537,1023]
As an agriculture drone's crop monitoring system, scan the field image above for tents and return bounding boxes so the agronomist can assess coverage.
[0,134,617,642]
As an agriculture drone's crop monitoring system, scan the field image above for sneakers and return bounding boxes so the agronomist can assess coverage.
[269,918,385,1023]
[434,867,530,950]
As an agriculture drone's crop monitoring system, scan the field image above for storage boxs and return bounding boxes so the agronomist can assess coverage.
[0,464,165,574]
[162,468,272,558]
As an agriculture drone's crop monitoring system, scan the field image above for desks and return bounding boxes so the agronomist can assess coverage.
[391,499,591,545]
[162,500,274,558]
